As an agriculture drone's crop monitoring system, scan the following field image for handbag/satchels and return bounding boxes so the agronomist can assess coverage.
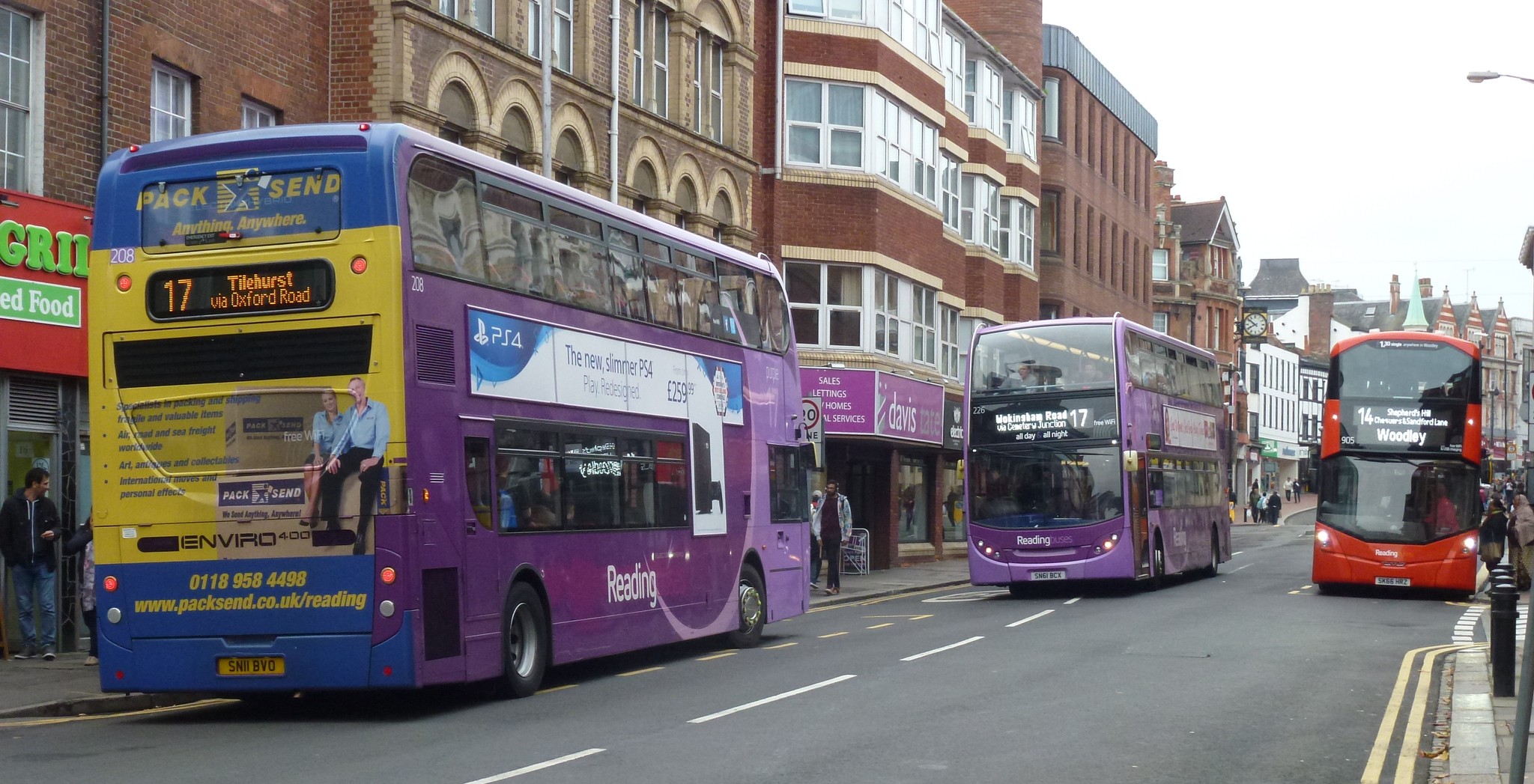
[1506,482,1513,490]
[1246,509,1251,517]
[1480,531,1501,562]
[1278,509,1282,518]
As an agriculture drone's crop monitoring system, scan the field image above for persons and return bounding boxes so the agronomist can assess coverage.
[62,505,99,666]
[464,440,686,530]
[1284,477,1293,502]
[1293,477,1301,503]
[898,483,916,531]
[810,480,852,595]
[1479,498,1507,594]
[0,467,61,663]
[299,378,390,556]
[994,359,1038,395]
[1416,470,1460,538]
[943,485,958,527]
[1082,359,1097,383]
[990,469,1036,505]
[1248,478,1281,525]
[1479,475,1526,527]
[1507,494,1534,590]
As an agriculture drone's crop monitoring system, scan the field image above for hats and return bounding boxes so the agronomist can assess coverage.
[813,490,822,498]
[1429,482,1446,492]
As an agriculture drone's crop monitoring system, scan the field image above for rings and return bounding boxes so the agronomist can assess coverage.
[363,464,366,467]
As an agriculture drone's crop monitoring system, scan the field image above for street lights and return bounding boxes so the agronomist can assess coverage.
[1473,331,1508,477]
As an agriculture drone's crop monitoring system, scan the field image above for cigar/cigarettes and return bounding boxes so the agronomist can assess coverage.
[43,535,44,538]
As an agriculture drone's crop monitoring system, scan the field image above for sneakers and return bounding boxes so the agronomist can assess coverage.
[14,645,38,659]
[42,645,56,659]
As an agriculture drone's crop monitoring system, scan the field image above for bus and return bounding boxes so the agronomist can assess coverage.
[86,123,817,698]
[955,311,1233,594]
[1304,331,1492,599]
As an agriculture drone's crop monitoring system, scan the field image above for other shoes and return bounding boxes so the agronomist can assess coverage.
[825,589,833,595]
[309,510,319,528]
[299,519,309,526]
[830,587,840,594]
[810,580,819,589]
[1485,589,1492,594]
[326,512,340,531]
[84,656,98,665]
[353,531,366,555]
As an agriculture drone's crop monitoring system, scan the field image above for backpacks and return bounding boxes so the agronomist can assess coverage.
[1256,497,1266,510]
[810,507,819,534]
